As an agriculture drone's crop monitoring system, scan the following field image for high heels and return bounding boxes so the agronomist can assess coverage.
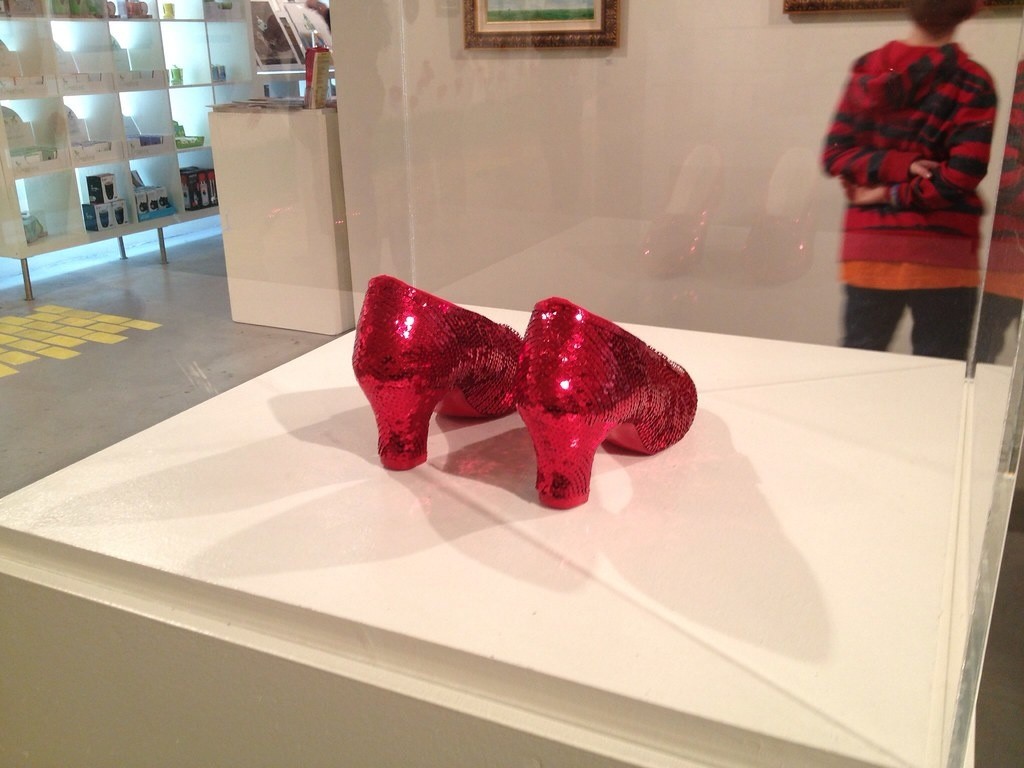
[512,295,698,509]
[351,273,526,472]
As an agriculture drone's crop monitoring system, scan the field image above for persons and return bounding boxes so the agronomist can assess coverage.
[822,0,998,362]
[975,59,1023,361]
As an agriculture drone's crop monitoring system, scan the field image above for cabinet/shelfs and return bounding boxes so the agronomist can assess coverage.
[209,108,355,335]
[0,0,336,301]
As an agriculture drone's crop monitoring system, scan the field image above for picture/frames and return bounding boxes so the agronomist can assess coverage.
[462,0,620,48]
